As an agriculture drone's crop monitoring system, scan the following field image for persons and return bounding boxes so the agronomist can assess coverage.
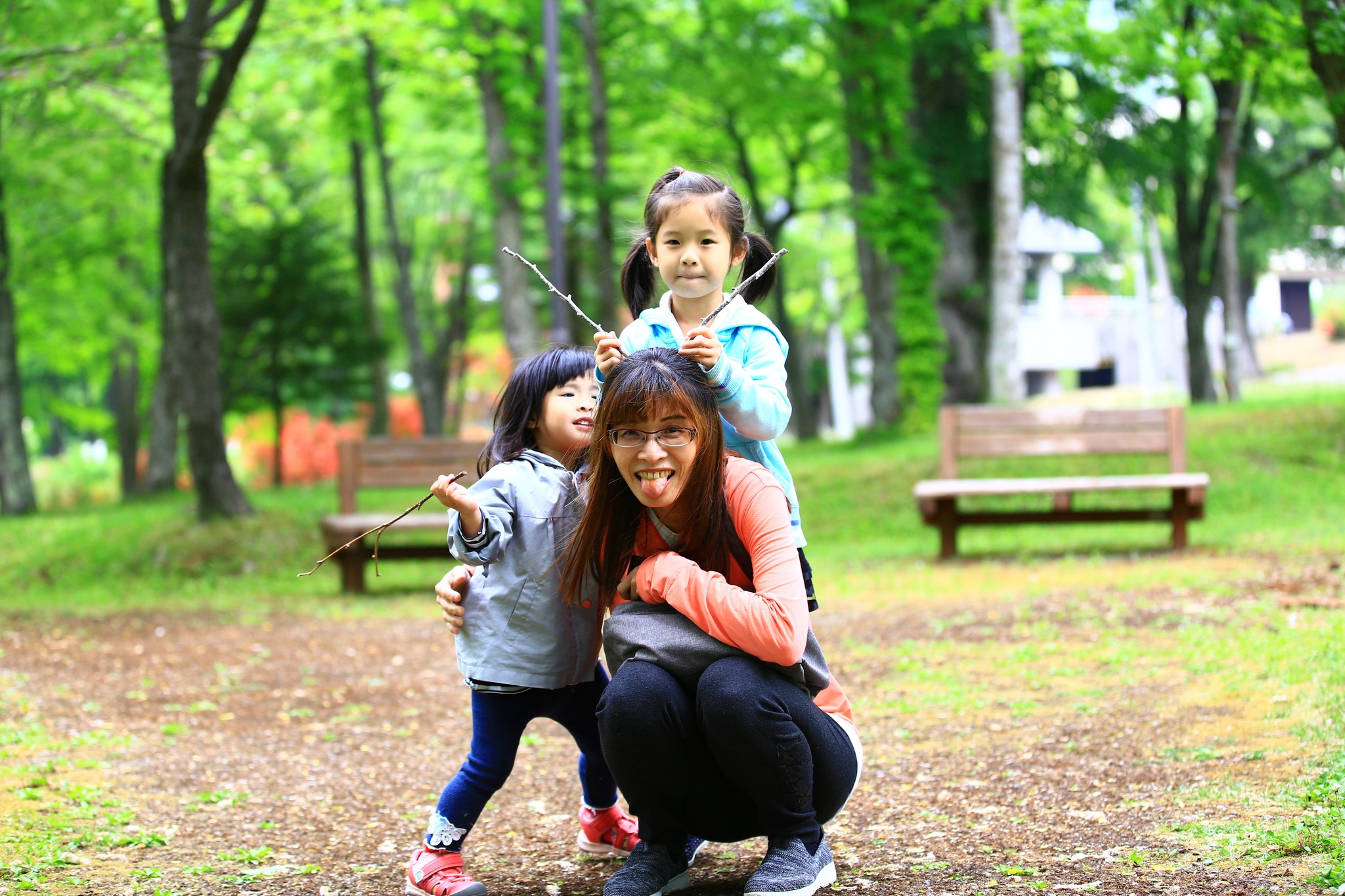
[397,346,641,895]
[430,346,863,896]
[590,164,819,610]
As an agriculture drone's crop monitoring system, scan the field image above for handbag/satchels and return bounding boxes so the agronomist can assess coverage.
[603,600,829,701]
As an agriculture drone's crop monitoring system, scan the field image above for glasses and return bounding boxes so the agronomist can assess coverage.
[607,426,697,447]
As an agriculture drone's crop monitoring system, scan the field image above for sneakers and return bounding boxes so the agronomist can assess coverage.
[603,841,690,896]
[686,836,709,867]
[576,796,643,857]
[404,849,488,896]
[744,838,836,896]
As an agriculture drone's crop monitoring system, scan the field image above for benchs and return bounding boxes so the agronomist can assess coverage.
[913,403,1209,555]
[318,440,493,595]
